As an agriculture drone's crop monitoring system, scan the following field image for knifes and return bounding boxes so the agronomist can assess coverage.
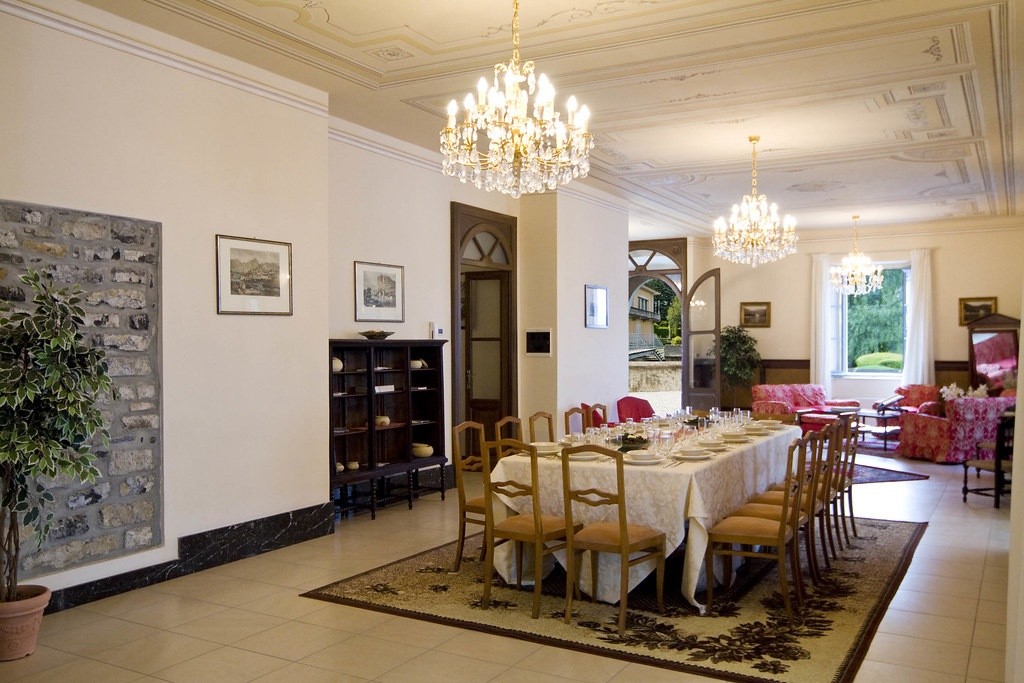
[662,461,684,469]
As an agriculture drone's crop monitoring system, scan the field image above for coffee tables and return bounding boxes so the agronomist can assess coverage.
[823,405,909,451]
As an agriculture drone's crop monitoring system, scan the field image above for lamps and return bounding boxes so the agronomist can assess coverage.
[712,134,797,269]
[829,215,885,297]
[440,1,595,198]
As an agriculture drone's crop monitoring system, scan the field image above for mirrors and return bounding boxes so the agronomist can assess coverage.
[966,312,1020,398]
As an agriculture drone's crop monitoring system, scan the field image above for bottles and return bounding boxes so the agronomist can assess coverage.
[411,361,422,368]
[347,461,359,470]
[337,463,344,472]
[375,416,391,427]
[332,357,344,372]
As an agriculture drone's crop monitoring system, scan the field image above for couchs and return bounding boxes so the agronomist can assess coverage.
[751,383,860,441]
[872,383,1017,464]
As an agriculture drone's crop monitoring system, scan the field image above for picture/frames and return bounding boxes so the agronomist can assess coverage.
[585,285,609,328]
[215,235,293,316]
[740,302,770,328]
[959,297,998,327]
[354,261,406,323]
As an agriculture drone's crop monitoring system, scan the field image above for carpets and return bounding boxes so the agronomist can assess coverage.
[805,460,929,485]
[803,438,899,457]
[297,513,929,683]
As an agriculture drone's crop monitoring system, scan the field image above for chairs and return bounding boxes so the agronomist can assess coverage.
[961,408,1016,509]
[452,397,860,636]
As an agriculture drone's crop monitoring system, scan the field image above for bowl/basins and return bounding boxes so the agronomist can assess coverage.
[741,425,766,431]
[756,420,783,425]
[626,449,657,460]
[529,434,571,451]
[412,445,434,458]
[679,447,705,456]
[358,331,396,340]
[572,452,598,456]
[720,431,747,439]
[698,441,724,447]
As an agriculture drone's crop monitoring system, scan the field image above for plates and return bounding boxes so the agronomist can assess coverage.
[561,440,572,446]
[722,436,750,442]
[703,446,727,451]
[669,451,715,460]
[520,447,565,456]
[744,430,769,436]
[764,424,788,430]
[557,452,600,461]
[623,455,667,464]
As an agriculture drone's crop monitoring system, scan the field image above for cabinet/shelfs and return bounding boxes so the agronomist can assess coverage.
[328,339,449,521]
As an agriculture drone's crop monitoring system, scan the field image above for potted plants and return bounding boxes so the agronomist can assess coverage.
[0,266,113,662]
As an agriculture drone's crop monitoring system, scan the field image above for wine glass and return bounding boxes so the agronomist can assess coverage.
[570,405,752,453]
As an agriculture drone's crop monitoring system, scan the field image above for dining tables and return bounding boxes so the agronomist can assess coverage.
[483,418,802,614]
